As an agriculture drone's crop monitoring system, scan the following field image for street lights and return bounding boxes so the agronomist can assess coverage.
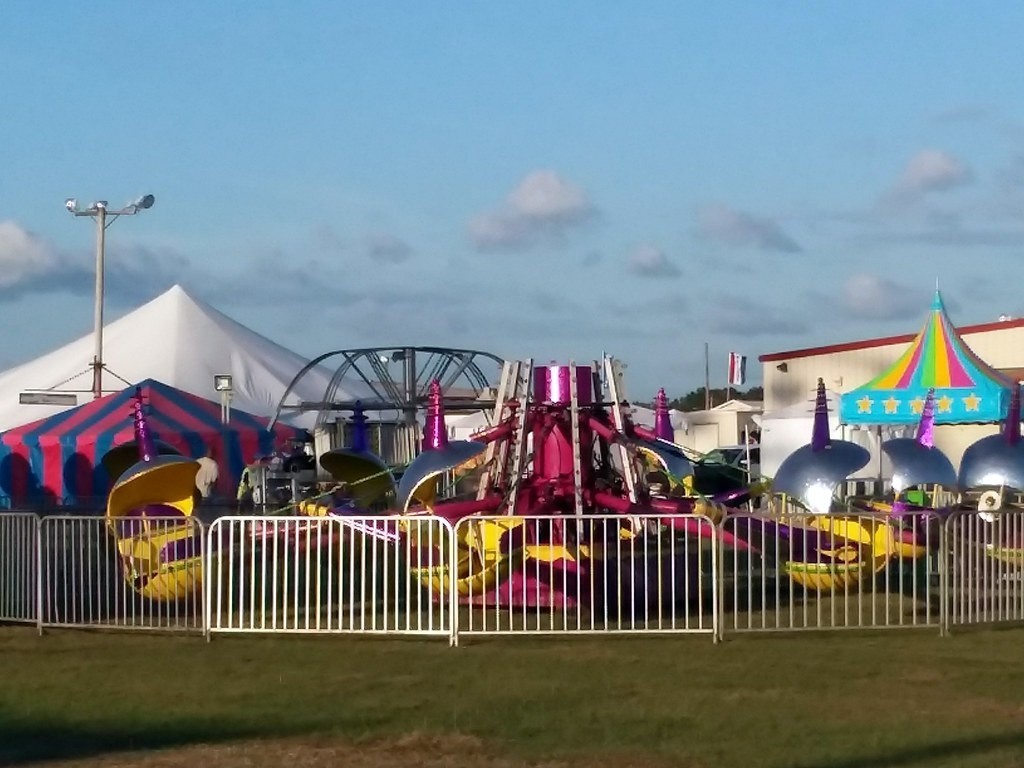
[65,194,156,400]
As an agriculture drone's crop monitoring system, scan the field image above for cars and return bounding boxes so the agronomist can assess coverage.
[703,443,760,480]
